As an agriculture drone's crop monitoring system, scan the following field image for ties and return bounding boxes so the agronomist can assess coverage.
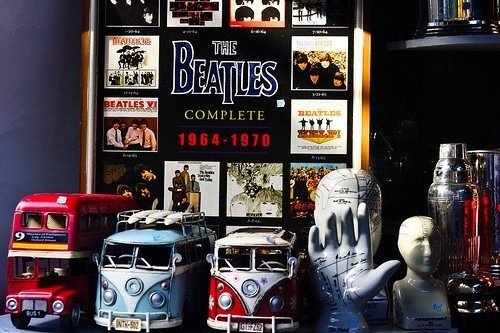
[142,131,145,147]
[115,129,118,142]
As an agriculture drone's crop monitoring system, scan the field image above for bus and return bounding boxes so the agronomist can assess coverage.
[93,210,218,333]
[4,193,139,333]
[206,226,300,333]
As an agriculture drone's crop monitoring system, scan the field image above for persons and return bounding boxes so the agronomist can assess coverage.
[106,118,157,151]
[392,216,452,330]
[117,164,164,211]
[173,165,200,213]
[293,53,346,89]
[307,168,401,333]
